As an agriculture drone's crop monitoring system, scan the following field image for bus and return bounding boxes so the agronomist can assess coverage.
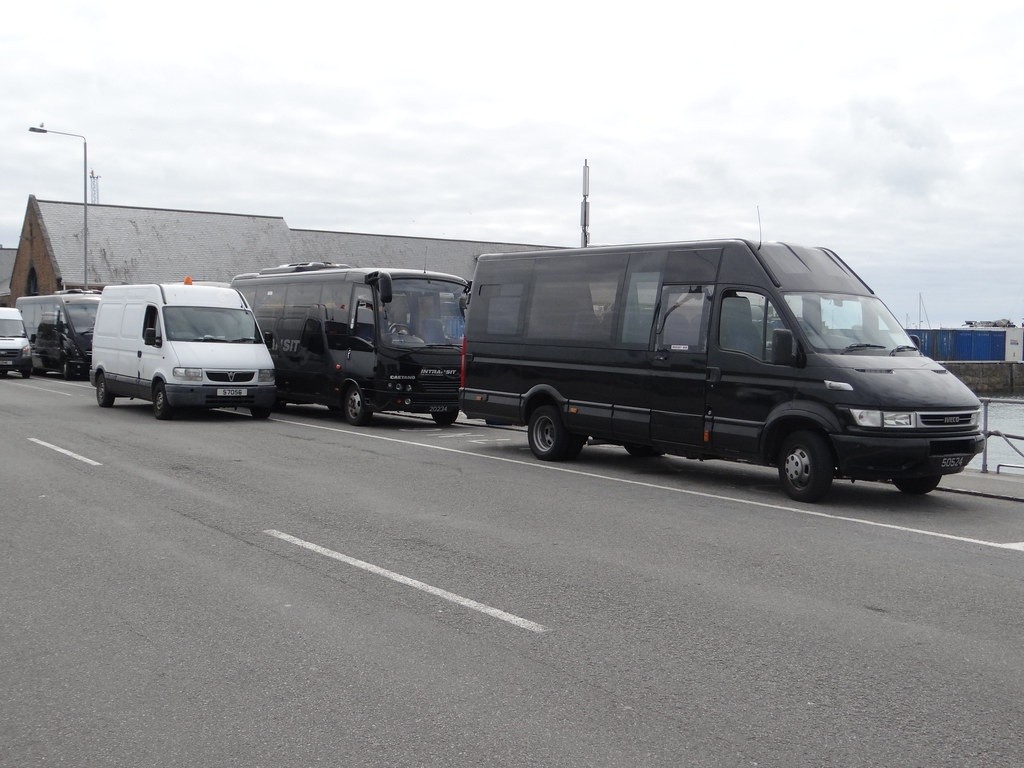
[230,258,472,428]
[16,289,103,380]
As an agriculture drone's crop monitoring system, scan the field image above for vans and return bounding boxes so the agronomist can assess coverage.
[0,307,37,382]
[88,276,277,420]
[460,230,988,505]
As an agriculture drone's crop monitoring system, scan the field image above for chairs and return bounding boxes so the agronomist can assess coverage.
[720,296,769,362]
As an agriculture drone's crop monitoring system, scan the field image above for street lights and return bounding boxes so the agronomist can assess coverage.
[29,127,88,288]
